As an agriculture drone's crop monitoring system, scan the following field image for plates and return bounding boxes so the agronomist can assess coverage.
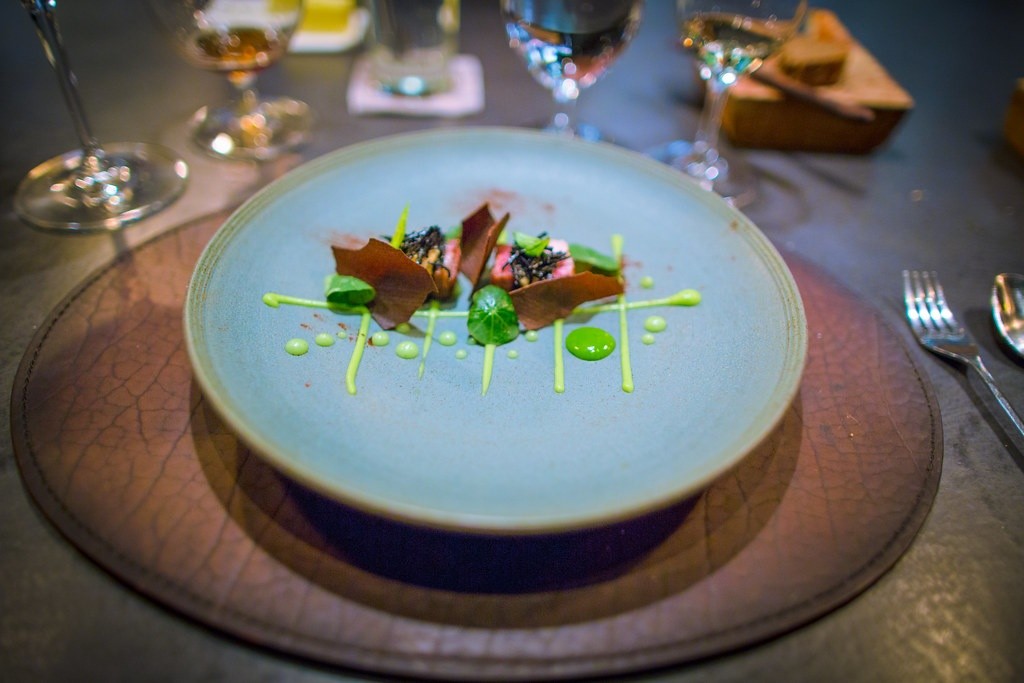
[185,126,807,535]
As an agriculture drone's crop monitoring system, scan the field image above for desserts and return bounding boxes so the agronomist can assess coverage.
[323,204,624,344]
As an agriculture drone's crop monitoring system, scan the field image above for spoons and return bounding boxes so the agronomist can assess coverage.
[992,273,1024,355]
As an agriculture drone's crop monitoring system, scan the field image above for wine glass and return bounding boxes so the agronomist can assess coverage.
[500,0,644,139]
[643,0,810,208]
[155,0,317,156]
[12,0,188,230]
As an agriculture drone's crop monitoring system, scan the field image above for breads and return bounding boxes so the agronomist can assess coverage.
[776,36,847,85]
[804,8,853,54]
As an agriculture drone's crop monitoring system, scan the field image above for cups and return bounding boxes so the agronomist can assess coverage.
[369,0,460,97]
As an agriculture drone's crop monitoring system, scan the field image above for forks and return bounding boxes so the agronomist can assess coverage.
[902,270,1024,460]
